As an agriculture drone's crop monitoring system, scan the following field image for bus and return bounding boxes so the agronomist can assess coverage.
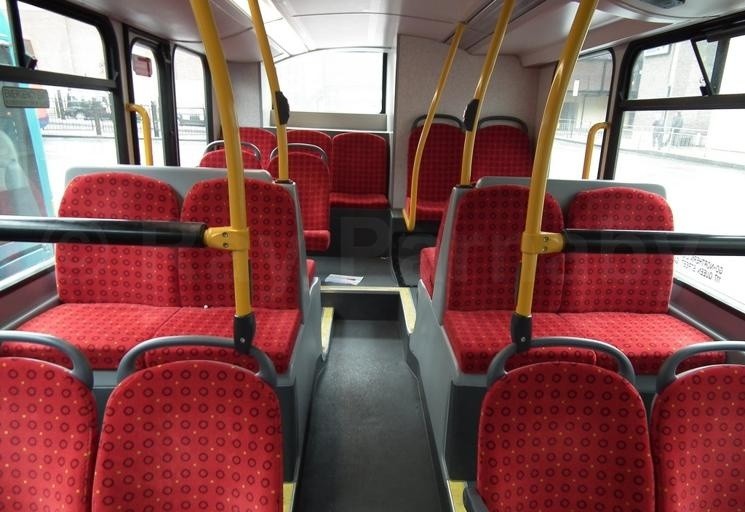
[0,10,56,282]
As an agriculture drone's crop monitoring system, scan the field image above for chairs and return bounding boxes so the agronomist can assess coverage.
[216,127,275,166]
[431,185,596,464]
[267,143,330,252]
[304,249,326,382]
[464,338,657,509]
[198,140,262,173]
[330,130,389,255]
[144,176,304,449]
[0,328,96,512]
[391,113,468,285]
[468,115,533,183]
[90,333,285,511]
[649,339,745,511]
[274,130,331,160]
[562,187,729,375]
[0,169,182,373]
[405,186,459,356]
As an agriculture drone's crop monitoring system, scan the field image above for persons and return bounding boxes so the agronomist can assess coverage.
[651,114,666,150]
[666,112,685,146]
